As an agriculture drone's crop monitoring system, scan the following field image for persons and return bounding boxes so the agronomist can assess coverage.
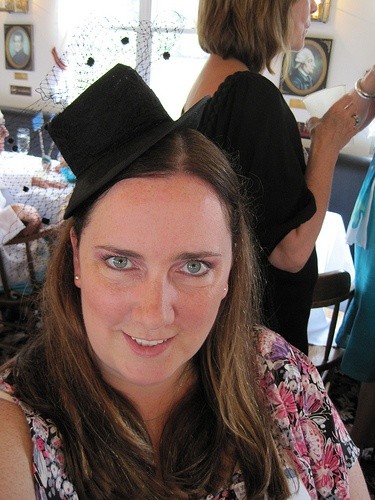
[180,0,375,359]
[0,122,371,500]
[0,109,69,287]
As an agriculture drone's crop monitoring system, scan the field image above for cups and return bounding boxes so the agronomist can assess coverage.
[17,128,30,156]
[303,85,347,119]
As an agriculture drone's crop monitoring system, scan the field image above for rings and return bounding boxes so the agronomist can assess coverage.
[352,114,360,126]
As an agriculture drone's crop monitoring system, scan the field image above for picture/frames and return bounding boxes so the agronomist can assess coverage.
[278,36,333,97]
[4,23,34,71]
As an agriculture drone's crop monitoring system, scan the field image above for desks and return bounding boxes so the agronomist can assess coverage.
[0,151,73,222]
[307,209,355,348]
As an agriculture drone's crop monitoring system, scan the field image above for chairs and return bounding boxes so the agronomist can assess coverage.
[307,271,355,386]
[0,221,61,363]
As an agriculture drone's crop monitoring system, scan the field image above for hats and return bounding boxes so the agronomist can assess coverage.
[44,64,214,219]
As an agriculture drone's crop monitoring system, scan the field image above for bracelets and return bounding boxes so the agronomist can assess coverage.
[354,77,375,99]
